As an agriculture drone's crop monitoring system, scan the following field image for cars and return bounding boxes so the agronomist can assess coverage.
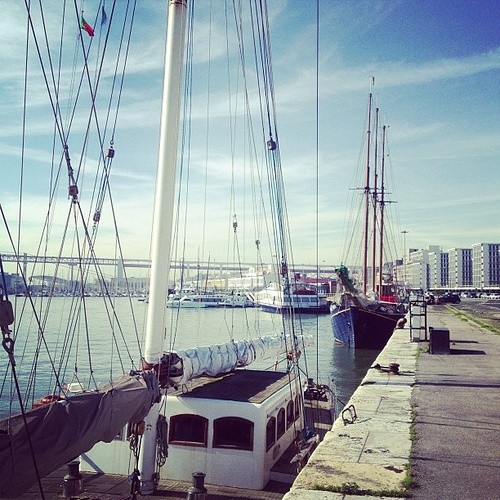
[436,293,462,306]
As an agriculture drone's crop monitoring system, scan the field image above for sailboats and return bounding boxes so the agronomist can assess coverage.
[329,92,398,347]
[0,1,341,500]
[165,248,253,309]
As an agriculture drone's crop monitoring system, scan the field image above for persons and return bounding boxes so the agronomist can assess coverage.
[406,292,410,303]
[428,293,435,304]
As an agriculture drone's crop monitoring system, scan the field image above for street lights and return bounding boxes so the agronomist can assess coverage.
[401,227,410,281]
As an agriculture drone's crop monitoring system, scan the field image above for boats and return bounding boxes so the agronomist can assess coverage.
[256,273,321,314]
